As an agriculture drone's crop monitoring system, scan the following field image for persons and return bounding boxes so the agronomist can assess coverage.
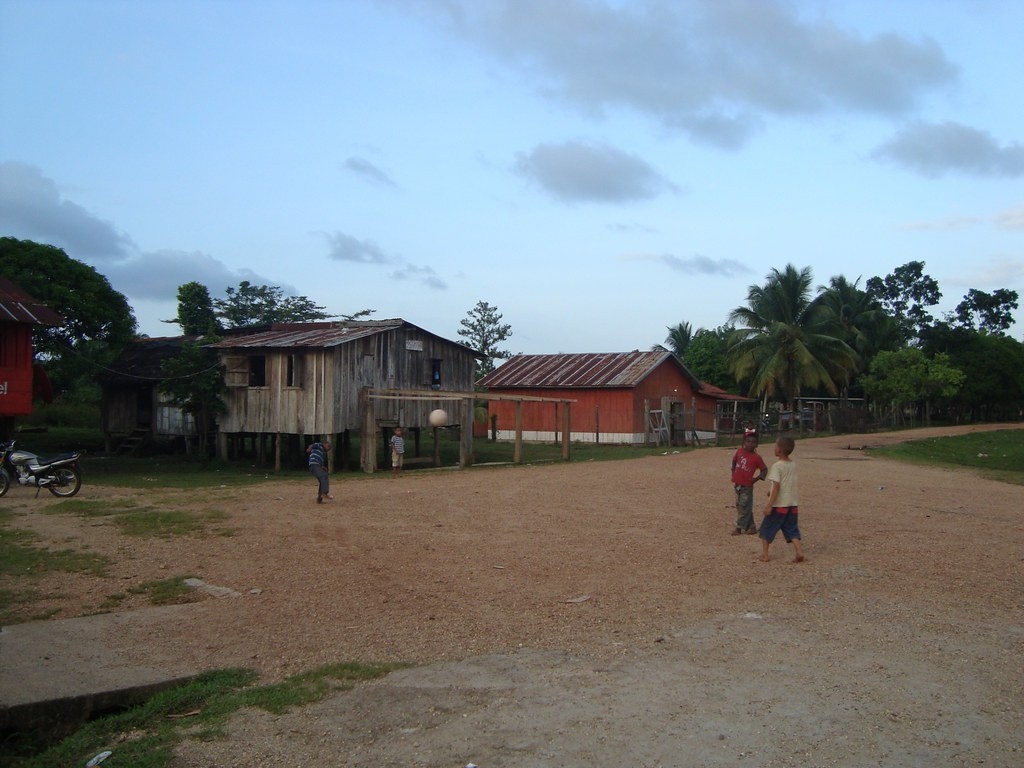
[758,436,805,564]
[389,425,406,479]
[731,433,768,536]
[742,420,757,449]
[306,441,333,505]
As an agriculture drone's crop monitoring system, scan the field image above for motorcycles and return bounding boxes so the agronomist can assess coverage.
[0,440,81,500]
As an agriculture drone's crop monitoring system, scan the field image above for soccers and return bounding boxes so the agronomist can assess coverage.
[429,409,447,427]
